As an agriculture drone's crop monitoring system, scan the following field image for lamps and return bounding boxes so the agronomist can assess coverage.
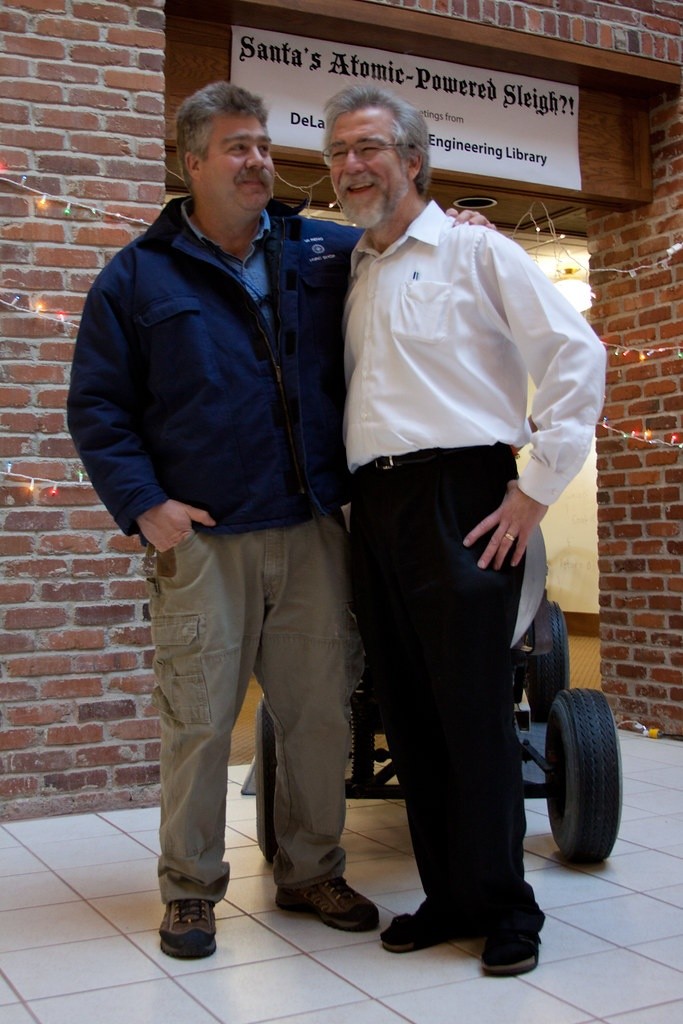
[553,268,593,313]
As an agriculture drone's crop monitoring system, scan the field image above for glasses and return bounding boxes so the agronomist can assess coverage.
[322,140,416,167]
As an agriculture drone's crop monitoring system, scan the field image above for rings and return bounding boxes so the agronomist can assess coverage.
[505,533,515,541]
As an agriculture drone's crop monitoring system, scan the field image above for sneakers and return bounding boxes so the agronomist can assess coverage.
[275,876,379,932]
[159,899,216,956]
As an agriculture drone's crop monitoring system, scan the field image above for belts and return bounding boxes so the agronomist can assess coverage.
[359,448,462,470]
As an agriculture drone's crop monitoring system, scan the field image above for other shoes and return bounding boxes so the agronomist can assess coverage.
[380,909,472,953]
[482,929,539,974]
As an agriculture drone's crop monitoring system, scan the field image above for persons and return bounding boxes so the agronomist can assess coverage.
[322,84,606,974]
[67,80,497,957]
